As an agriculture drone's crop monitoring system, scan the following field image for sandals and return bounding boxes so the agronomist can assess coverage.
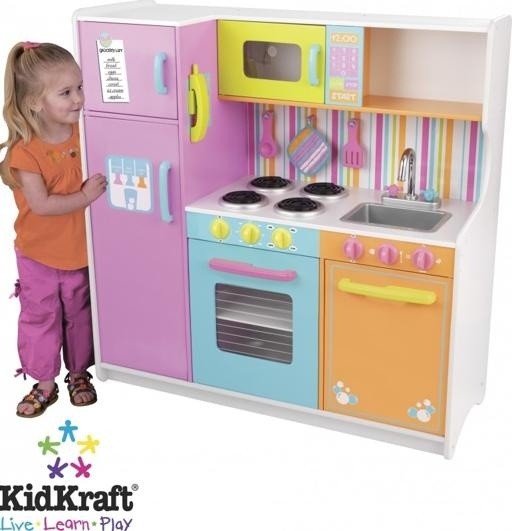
[17,383,59,417]
[64,371,97,406]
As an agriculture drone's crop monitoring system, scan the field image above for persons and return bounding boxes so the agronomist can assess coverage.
[0,41,108,419]
[73,0,512,457]
